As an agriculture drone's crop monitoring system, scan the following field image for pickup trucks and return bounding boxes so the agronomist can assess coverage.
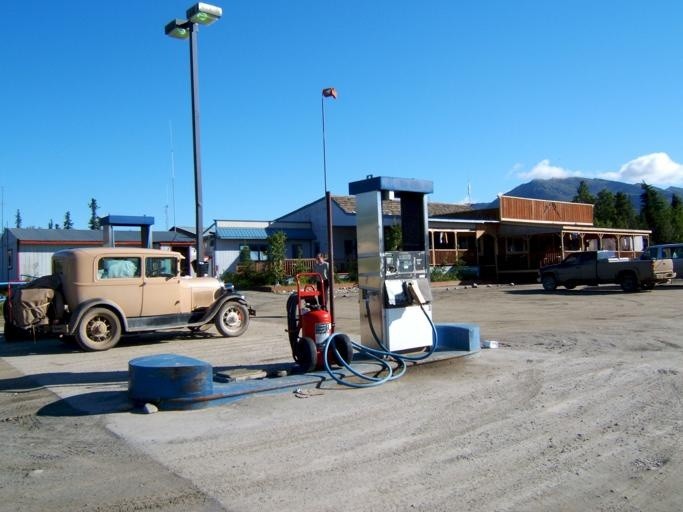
[540,251,676,292]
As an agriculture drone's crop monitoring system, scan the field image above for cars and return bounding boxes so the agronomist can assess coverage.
[51,247,250,352]
[644,242,683,277]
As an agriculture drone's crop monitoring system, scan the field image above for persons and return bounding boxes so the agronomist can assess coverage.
[313,252,330,312]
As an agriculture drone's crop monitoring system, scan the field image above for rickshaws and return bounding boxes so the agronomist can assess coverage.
[289,272,353,373]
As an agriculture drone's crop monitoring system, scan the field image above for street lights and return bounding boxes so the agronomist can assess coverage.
[321,87,337,192]
[165,2,222,276]
